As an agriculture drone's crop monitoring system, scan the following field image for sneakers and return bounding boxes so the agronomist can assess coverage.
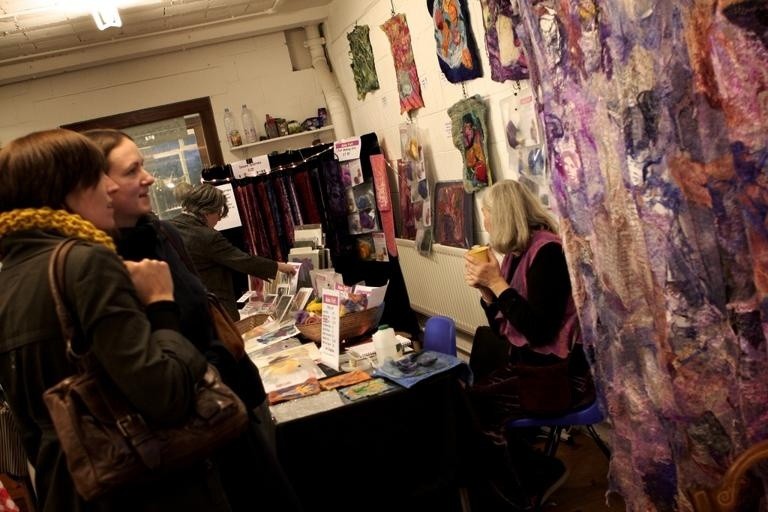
[489,450,570,510]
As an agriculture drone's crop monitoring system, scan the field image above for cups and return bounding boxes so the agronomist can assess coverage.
[465,245,490,265]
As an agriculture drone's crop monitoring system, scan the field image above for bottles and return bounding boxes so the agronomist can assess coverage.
[242,103,258,144]
[221,106,243,147]
[370,323,398,364]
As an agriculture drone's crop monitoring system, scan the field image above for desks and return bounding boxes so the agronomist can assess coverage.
[228,302,475,510]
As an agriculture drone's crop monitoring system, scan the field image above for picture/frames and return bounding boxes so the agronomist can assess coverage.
[431,179,473,250]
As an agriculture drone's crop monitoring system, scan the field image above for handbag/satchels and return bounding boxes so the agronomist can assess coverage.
[511,362,576,414]
[204,290,244,363]
[43,335,248,501]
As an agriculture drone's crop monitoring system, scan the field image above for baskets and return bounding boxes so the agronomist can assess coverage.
[237,314,270,341]
[297,302,386,344]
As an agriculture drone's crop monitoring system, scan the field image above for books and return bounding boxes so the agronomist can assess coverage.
[280,223,333,290]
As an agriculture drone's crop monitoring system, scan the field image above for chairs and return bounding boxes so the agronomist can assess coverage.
[423,317,457,358]
[467,324,508,372]
[511,318,618,484]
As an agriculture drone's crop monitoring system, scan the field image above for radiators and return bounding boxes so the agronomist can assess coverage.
[393,238,490,338]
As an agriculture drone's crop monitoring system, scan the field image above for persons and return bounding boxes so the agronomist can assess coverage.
[455,177,597,512]
[2,128,209,511]
[165,184,298,285]
[80,127,280,511]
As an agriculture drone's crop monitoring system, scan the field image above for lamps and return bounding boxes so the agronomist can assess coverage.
[89,2,123,32]
